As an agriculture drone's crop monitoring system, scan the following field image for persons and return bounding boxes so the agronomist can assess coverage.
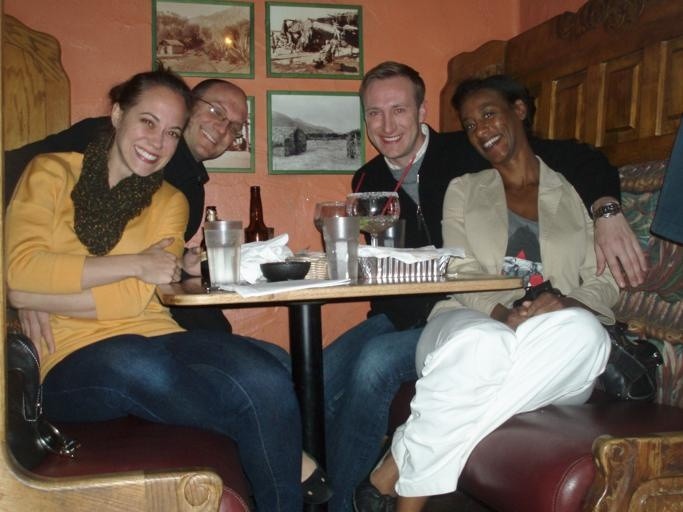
[322,60,649,469]
[271,14,358,68]
[352,74,621,512]
[16,79,293,374]
[2,71,333,512]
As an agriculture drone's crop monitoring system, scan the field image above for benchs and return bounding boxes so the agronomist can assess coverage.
[0,7,262,512]
[372,0,681,512]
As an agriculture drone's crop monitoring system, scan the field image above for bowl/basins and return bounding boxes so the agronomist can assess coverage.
[260,261,310,282]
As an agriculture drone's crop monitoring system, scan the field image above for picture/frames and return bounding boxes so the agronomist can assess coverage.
[204,94,258,176]
[149,1,254,82]
[265,89,367,177]
[264,0,363,81]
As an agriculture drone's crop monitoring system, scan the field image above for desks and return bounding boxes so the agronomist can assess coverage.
[156,264,535,510]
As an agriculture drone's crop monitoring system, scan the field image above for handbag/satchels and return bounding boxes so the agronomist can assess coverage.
[5,332,81,472]
[512,278,664,405]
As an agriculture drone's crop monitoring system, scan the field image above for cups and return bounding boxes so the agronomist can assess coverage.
[320,217,360,283]
[243,228,274,242]
[378,220,406,249]
[203,220,241,282]
[313,200,346,234]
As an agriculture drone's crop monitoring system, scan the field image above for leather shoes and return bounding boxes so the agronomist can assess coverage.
[299,449,335,506]
[350,427,399,511]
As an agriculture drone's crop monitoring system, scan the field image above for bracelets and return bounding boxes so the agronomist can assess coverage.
[592,201,622,223]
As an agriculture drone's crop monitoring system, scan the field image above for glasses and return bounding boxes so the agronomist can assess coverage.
[195,95,243,140]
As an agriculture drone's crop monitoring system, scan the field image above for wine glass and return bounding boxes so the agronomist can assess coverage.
[347,192,402,249]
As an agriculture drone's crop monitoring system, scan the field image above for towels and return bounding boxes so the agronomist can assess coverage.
[211,276,354,299]
[232,232,296,288]
[352,240,467,266]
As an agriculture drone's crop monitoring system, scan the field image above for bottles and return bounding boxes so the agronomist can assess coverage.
[245,182,268,242]
[200,205,221,285]
